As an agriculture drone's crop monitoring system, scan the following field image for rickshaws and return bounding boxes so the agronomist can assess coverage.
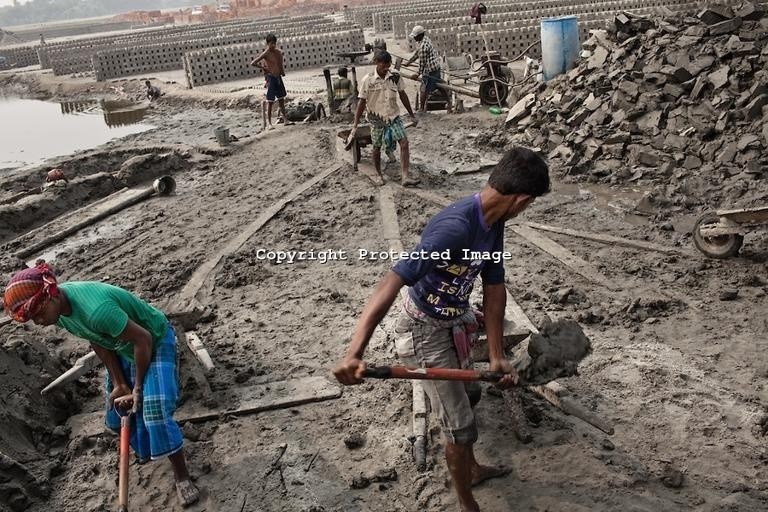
[275,53,515,171]
[692,206,768,258]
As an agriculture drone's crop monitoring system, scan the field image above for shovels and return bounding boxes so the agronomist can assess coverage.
[362,362,566,390]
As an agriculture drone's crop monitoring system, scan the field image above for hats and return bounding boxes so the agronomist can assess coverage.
[409,25,424,38]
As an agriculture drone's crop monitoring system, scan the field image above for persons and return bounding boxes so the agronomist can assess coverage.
[331,146,552,512]
[4,262,200,506]
[400,25,452,115]
[145,80,160,102]
[250,34,294,130]
[351,49,421,187]
[332,67,354,109]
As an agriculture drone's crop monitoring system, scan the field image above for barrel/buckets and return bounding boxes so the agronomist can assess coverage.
[153,175,177,196]
[214,127,230,146]
